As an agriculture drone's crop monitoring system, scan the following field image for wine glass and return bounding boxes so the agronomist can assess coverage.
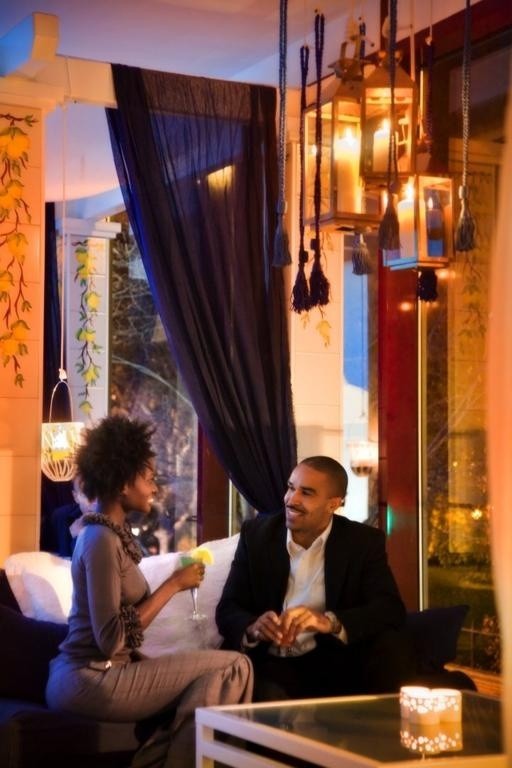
[179,554,208,622]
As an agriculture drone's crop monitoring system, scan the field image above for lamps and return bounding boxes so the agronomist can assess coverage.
[40,102,85,484]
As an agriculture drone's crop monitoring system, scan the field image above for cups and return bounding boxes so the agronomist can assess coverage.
[399,685,463,727]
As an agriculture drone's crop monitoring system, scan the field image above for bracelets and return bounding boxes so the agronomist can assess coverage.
[323,611,342,640]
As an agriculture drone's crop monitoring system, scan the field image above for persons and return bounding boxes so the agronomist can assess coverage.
[42,411,254,767]
[213,452,408,708]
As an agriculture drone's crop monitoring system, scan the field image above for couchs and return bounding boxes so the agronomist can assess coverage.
[1,529,248,766]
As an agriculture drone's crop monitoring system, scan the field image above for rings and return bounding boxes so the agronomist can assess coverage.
[253,630,260,639]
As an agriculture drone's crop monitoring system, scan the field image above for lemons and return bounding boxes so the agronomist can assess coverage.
[191,549,213,565]
